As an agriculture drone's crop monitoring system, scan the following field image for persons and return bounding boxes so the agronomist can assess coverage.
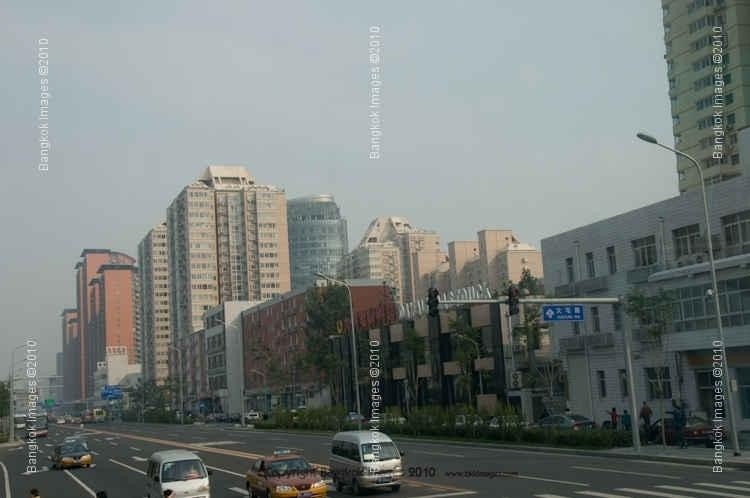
[663,404,689,449]
[639,400,653,428]
[605,407,619,433]
[29,488,41,498]
[621,408,633,431]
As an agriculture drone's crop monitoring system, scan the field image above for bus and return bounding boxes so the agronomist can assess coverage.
[14,412,48,438]
[81,409,105,424]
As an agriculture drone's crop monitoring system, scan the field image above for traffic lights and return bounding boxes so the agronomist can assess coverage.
[427,288,439,318]
[508,285,520,316]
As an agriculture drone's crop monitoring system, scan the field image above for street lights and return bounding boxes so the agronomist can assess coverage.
[214,318,245,428]
[636,133,742,457]
[251,369,267,417]
[167,346,184,424]
[9,344,40,444]
[454,333,484,395]
[313,273,361,430]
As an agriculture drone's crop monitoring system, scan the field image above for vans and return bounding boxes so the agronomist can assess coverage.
[145,450,213,498]
[329,430,405,496]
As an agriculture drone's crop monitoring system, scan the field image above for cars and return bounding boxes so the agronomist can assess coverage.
[51,440,92,470]
[205,407,406,425]
[56,415,82,425]
[440,414,727,448]
[245,450,328,498]
